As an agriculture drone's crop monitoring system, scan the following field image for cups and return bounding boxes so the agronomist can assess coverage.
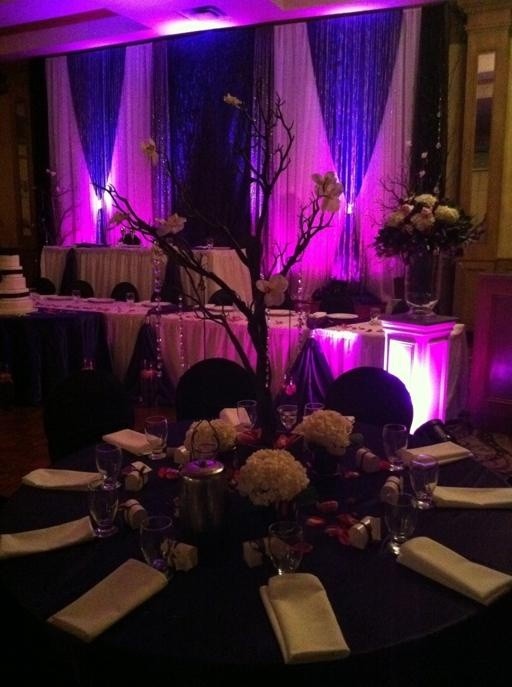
[304,403,325,416]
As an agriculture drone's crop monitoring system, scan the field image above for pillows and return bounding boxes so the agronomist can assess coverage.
[21,467,105,492]
[397,535,512,608]
[48,557,166,645]
[0,516,95,558]
[429,483,512,512]
[103,428,163,457]
[258,569,351,668]
[403,439,474,470]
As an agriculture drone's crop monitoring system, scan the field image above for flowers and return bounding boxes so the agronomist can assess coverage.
[292,408,357,459]
[182,418,239,462]
[232,449,312,515]
[88,67,355,403]
[374,53,487,261]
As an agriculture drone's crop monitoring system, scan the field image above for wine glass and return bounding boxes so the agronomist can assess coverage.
[383,423,408,463]
[268,521,303,575]
[141,515,174,582]
[88,478,118,538]
[277,405,297,432]
[410,454,438,509]
[145,415,168,460]
[96,442,122,490]
[238,400,257,431]
[385,492,418,554]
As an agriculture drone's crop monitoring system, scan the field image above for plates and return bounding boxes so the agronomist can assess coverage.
[44,289,360,320]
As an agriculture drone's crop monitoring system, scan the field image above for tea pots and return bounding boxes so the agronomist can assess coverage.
[181,460,226,535]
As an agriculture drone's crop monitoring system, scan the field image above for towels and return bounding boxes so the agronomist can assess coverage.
[236,536,297,571]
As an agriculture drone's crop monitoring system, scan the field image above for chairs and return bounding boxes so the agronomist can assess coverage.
[39,369,118,464]
